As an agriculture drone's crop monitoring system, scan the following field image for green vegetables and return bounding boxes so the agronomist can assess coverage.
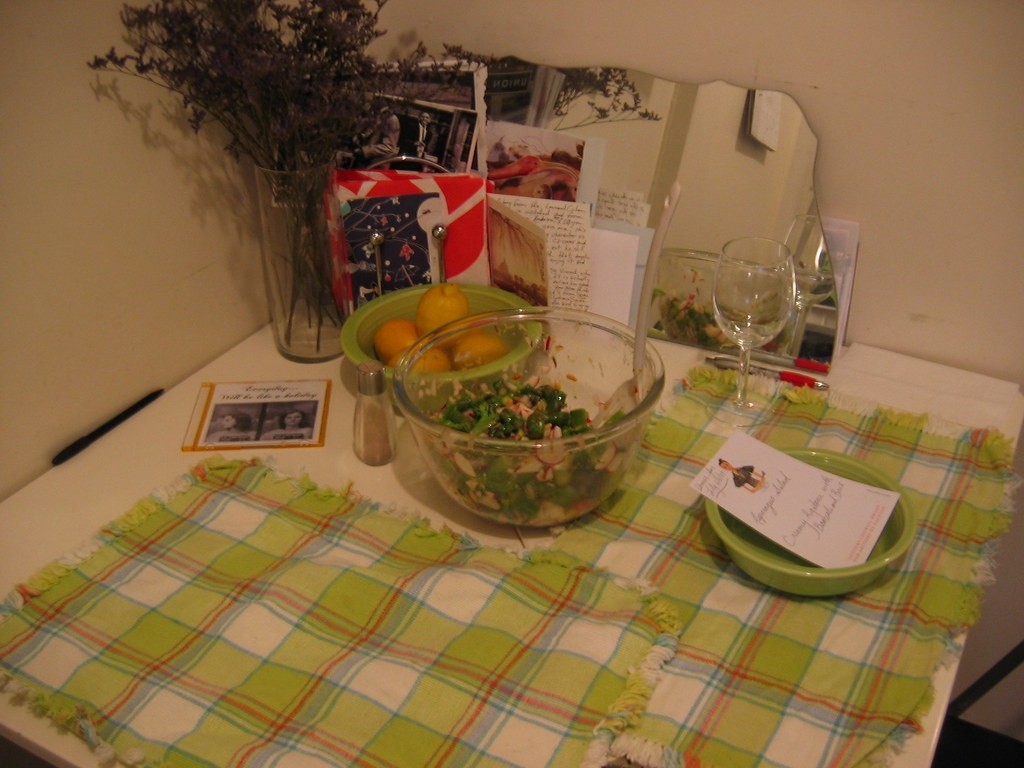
[429,381,628,523]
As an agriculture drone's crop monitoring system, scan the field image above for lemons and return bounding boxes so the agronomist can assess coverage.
[374,282,509,374]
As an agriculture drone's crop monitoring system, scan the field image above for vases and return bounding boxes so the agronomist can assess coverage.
[256,153,351,364]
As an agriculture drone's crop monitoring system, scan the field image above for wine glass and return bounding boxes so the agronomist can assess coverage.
[779,215,835,362]
[701,236,799,427]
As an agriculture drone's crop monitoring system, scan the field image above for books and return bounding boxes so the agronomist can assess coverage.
[325,167,491,322]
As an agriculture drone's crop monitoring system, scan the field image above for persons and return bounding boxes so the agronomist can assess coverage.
[344,103,432,172]
[720,459,766,492]
[263,411,313,441]
[206,412,255,443]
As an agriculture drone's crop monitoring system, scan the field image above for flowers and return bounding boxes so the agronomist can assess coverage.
[81,1,516,353]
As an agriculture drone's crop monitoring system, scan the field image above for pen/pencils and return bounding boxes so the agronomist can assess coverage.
[705,355,830,391]
[719,345,831,375]
[51,387,164,464]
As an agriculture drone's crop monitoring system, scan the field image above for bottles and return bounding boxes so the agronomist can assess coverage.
[351,366,399,466]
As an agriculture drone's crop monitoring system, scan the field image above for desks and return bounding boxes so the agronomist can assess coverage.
[3,287,1024,768]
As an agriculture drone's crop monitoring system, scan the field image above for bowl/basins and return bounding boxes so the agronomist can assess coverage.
[703,443,919,596]
[340,282,543,410]
[656,245,748,352]
[392,304,665,529]
